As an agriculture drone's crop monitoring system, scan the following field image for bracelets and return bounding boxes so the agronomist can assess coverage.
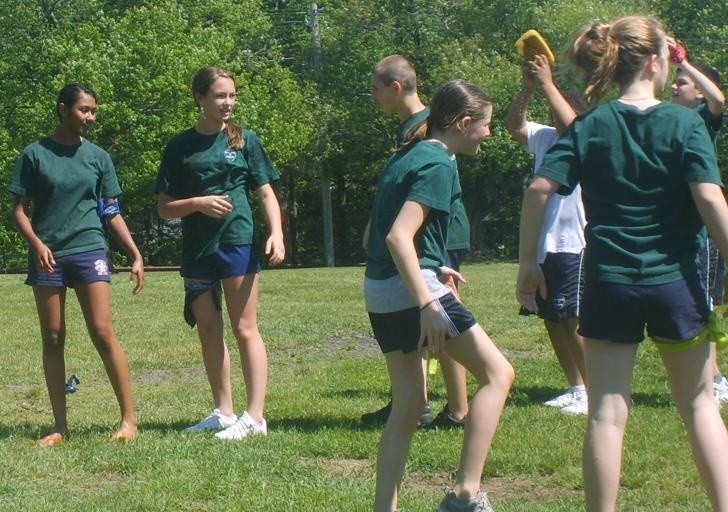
[418,297,435,314]
[667,43,685,65]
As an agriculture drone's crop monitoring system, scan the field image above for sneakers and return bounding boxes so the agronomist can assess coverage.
[361,401,432,428]
[544,389,573,408]
[560,392,589,416]
[185,409,237,433]
[427,407,466,431]
[435,491,494,512]
[215,411,268,440]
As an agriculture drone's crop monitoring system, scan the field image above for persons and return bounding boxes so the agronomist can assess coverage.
[152,63,286,443]
[513,13,726,511]
[361,53,470,432]
[661,34,726,409]
[8,81,145,450]
[502,54,589,416]
[361,77,515,512]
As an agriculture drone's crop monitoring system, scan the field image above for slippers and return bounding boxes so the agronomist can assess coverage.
[37,433,69,449]
[112,429,137,441]
